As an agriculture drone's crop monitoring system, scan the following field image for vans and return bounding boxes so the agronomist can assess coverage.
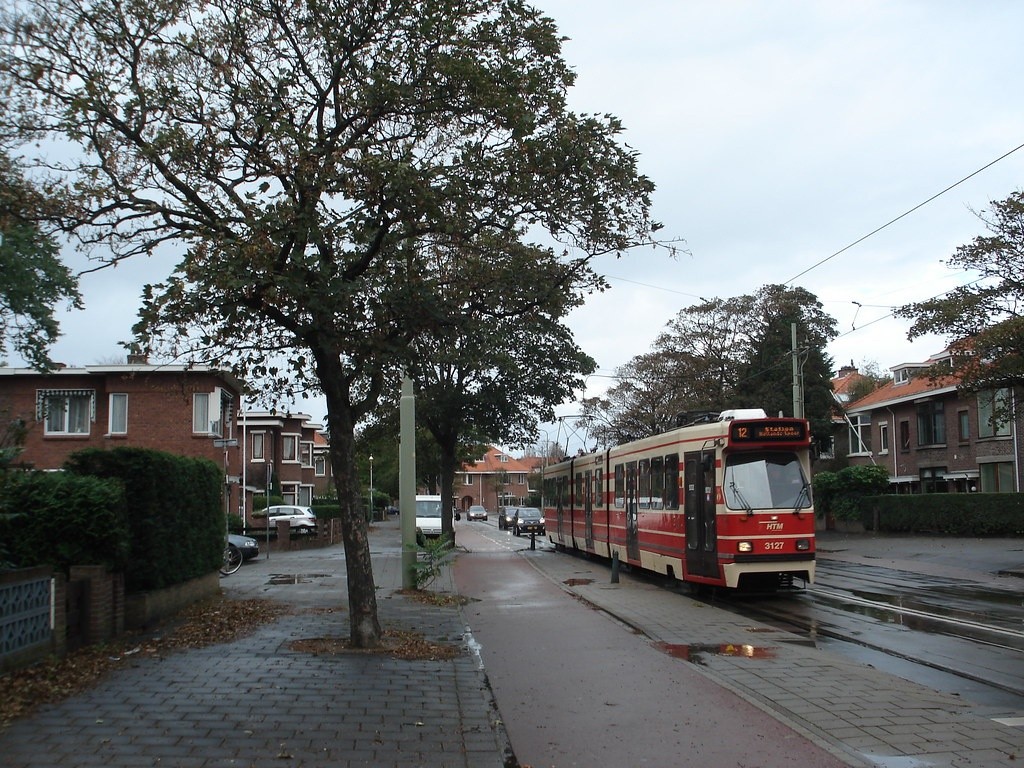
[416,494,461,547]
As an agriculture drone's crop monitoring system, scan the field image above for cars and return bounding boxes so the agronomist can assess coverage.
[498,506,520,530]
[466,505,488,522]
[223,532,259,564]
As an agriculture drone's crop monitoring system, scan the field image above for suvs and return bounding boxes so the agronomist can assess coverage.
[252,505,318,534]
[512,507,545,536]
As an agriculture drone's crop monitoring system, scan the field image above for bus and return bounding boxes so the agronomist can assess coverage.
[540,409,816,597]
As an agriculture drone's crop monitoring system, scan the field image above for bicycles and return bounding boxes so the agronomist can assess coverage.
[219,547,243,575]
